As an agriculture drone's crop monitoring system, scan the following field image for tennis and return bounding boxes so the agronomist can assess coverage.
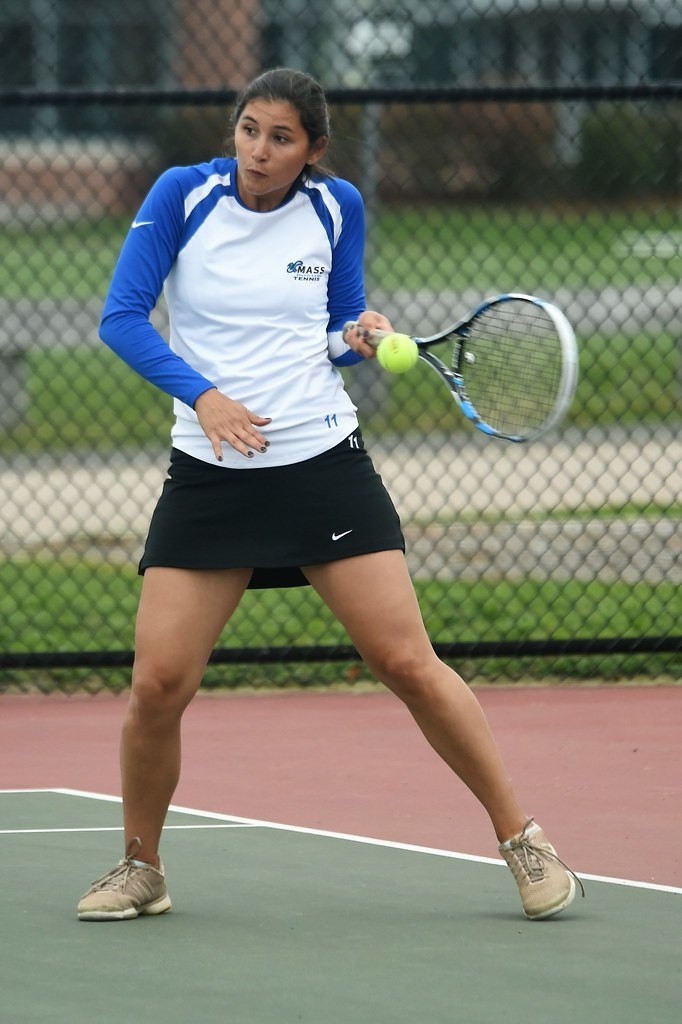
[376,333,419,375]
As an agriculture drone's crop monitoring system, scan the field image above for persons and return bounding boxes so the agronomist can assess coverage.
[76,69,576,922]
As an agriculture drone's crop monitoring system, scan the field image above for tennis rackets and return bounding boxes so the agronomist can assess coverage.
[341,292,580,444]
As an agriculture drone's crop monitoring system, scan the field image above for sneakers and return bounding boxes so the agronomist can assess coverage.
[75,837,171,919]
[498,814,584,920]
